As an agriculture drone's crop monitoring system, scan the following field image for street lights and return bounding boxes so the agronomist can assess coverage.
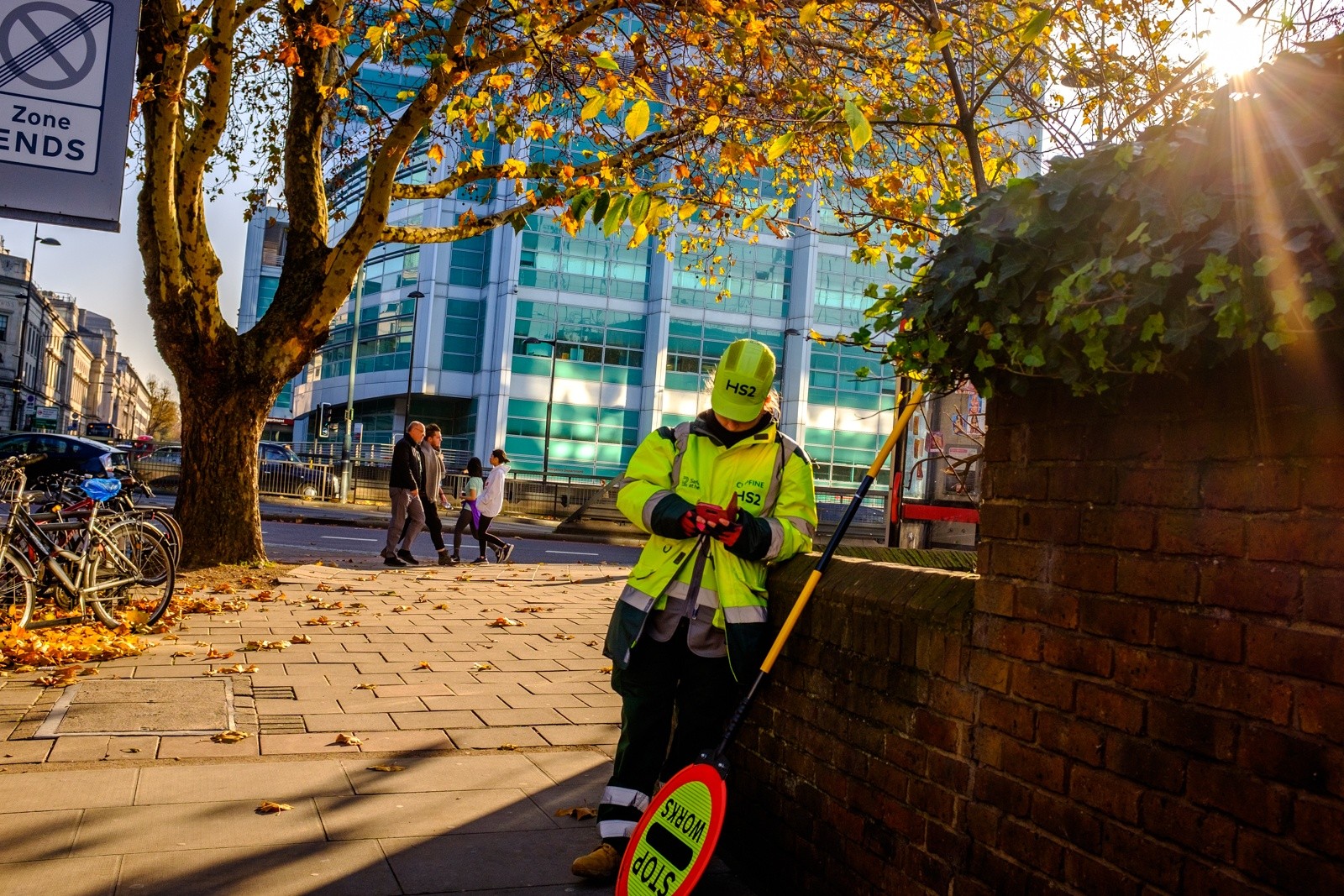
[522,337,558,487]
[10,223,63,434]
[28,333,76,430]
[777,327,802,433]
[404,273,424,436]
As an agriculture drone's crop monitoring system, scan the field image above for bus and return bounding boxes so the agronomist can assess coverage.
[86,422,122,443]
[136,435,155,459]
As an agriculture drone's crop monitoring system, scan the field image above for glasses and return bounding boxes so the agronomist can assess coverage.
[414,427,426,435]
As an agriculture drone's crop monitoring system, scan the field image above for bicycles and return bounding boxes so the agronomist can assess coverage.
[0,453,182,637]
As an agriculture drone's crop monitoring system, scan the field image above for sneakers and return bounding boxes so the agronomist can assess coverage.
[503,543,515,562]
[469,557,489,565]
[450,555,461,562]
[495,548,503,563]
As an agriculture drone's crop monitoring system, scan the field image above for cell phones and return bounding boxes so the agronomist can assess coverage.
[695,492,739,524]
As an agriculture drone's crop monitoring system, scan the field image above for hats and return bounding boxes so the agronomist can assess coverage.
[711,338,777,422]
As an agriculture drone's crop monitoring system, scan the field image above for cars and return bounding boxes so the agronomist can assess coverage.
[255,442,339,502]
[0,432,129,491]
[139,446,182,467]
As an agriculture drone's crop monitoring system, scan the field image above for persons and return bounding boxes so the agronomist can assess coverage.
[470,449,514,563]
[572,339,817,879]
[450,456,502,563]
[969,395,979,415]
[954,415,963,433]
[382,420,427,566]
[971,416,979,433]
[381,423,460,566]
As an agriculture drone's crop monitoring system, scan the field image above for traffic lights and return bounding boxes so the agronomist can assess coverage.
[318,402,333,438]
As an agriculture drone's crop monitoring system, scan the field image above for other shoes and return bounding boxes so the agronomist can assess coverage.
[437,556,456,566]
[397,549,419,565]
[380,549,397,559]
[383,557,406,567]
[570,841,621,878]
